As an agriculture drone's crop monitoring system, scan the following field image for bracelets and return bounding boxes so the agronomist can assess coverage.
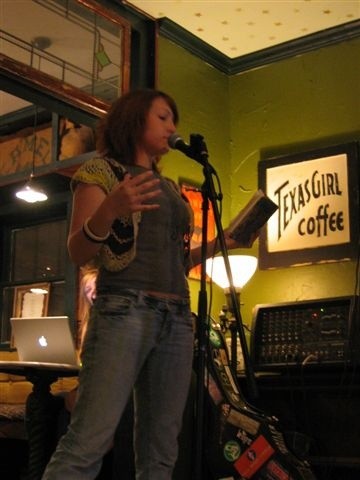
[82,217,110,243]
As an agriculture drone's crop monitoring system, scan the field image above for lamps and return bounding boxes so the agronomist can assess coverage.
[12,35,53,206]
[203,255,258,380]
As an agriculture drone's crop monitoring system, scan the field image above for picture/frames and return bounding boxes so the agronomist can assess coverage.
[256,139,359,272]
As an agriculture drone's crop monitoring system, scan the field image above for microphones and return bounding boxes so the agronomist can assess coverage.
[167,134,216,175]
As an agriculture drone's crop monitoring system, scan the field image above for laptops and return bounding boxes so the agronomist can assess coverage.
[10,316,77,365]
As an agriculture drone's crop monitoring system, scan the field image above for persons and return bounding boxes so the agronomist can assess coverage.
[39,86,267,480]
[56,264,99,444]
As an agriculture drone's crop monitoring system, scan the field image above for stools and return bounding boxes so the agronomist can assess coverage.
[0,360,82,480]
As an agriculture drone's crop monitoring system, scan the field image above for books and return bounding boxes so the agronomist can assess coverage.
[227,190,279,245]
